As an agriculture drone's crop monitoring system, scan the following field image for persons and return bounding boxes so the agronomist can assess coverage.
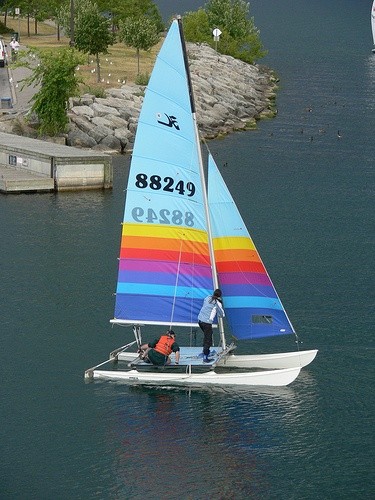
[198,289,225,362]
[9,37,20,63]
[140,330,180,365]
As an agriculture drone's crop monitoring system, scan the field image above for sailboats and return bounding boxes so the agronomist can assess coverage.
[84,14,318,386]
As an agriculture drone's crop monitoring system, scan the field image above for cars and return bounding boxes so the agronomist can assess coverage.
[0,40,8,67]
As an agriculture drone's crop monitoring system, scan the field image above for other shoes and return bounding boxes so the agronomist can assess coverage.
[139,353,144,360]
[144,357,149,363]
[203,355,214,363]
